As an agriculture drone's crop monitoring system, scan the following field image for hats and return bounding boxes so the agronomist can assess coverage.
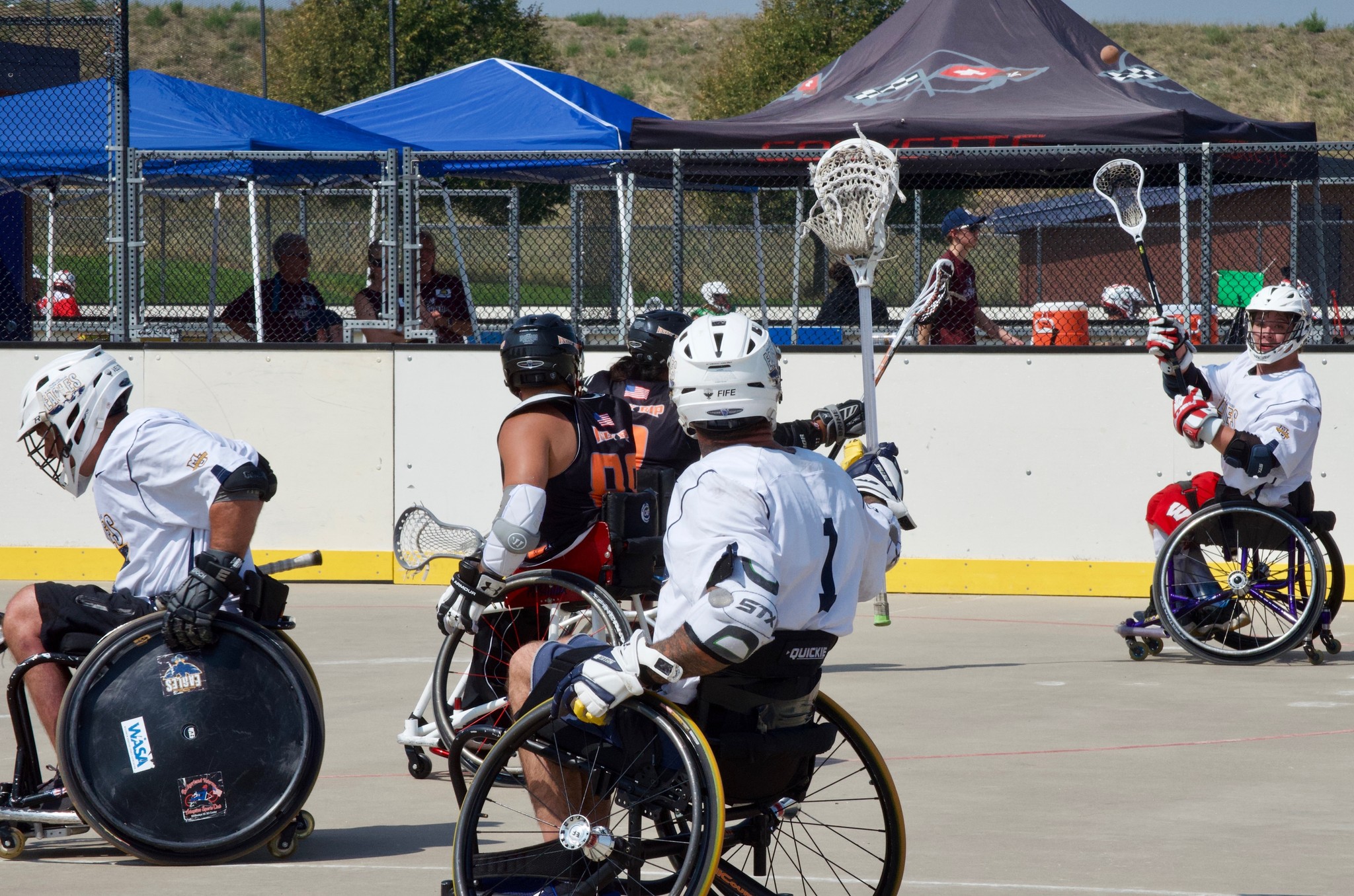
[942,207,987,235]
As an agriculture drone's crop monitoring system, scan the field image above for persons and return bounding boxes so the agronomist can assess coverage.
[1,207,1153,895]
[1123,282,1324,641]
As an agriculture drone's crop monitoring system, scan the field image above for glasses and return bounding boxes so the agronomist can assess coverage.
[956,223,981,233]
[288,252,308,260]
[373,259,389,268]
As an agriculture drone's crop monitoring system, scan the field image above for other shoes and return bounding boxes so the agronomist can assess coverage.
[37,763,75,811]
[534,875,621,896]
[455,717,501,748]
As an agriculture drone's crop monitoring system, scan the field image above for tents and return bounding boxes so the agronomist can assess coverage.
[2,0,1328,345]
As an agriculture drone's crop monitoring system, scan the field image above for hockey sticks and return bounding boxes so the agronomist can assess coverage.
[795,139,908,626]
[1093,159,1192,398]
[0,550,322,666]
[392,501,487,580]
[826,258,956,459]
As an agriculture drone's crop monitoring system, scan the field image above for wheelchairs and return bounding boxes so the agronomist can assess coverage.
[440,625,907,896]
[396,468,679,788]
[0,548,327,867]
[1113,480,1347,667]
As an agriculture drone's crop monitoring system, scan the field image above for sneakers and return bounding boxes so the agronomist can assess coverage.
[1132,606,1198,637]
[1192,599,1250,636]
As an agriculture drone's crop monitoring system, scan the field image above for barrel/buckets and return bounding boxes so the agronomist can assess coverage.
[1162,304,1217,345]
[1033,301,1089,345]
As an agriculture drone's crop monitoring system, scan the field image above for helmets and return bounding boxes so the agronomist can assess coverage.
[702,281,732,313]
[499,313,582,390]
[1243,285,1313,364]
[644,297,664,312]
[32,264,46,279]
[690,307,716,316]
[1280,278,1313,304]
[16,345,134,498]
[52,270,76,295]
[1100,284,1148,321]
[626,309,694,376]
[667,311,783,440]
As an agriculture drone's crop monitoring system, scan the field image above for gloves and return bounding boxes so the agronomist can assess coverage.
[436,556,507,634]
[547,628,684,731]
[1173,384,1223,449]
[157,547,245,657]
[846,442,918,531]
[1146,311,1197,376]
[811,399,867,447]
